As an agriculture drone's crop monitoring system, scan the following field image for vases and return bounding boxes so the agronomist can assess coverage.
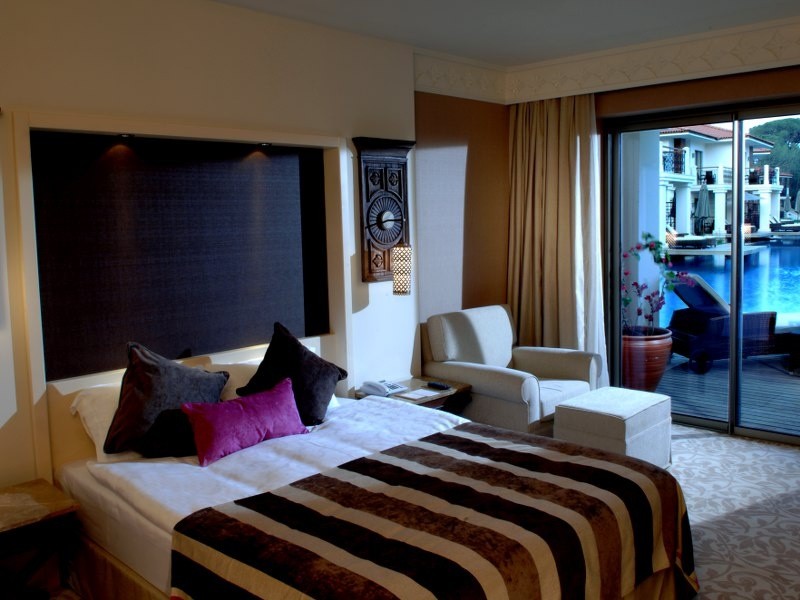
[621,326,673,392]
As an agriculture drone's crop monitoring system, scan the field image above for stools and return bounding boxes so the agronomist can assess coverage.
[552,386,675,472]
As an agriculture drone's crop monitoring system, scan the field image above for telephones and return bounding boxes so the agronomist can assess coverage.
[361,378,409,397]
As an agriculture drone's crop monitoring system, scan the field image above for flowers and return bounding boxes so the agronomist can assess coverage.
[618,233,697,337]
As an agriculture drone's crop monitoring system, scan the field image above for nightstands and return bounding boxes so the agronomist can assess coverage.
[354,374,474,418]
[0,477,78,537]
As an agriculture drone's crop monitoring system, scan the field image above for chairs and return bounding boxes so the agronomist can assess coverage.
[770,213,800,233]
[666,225,723,249]
[663,271,799,375]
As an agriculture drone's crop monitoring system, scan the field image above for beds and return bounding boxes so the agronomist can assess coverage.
[50,334,700,600]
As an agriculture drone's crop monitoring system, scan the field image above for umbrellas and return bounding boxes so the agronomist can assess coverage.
[694,181,711,237]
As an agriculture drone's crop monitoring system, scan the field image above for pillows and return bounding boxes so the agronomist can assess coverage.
[101,340,231,458]
[234,320,347,427]
[69,381,141,463]
[179,377,311,468]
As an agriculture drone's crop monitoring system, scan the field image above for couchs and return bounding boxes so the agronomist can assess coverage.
[420,302,603,440]
